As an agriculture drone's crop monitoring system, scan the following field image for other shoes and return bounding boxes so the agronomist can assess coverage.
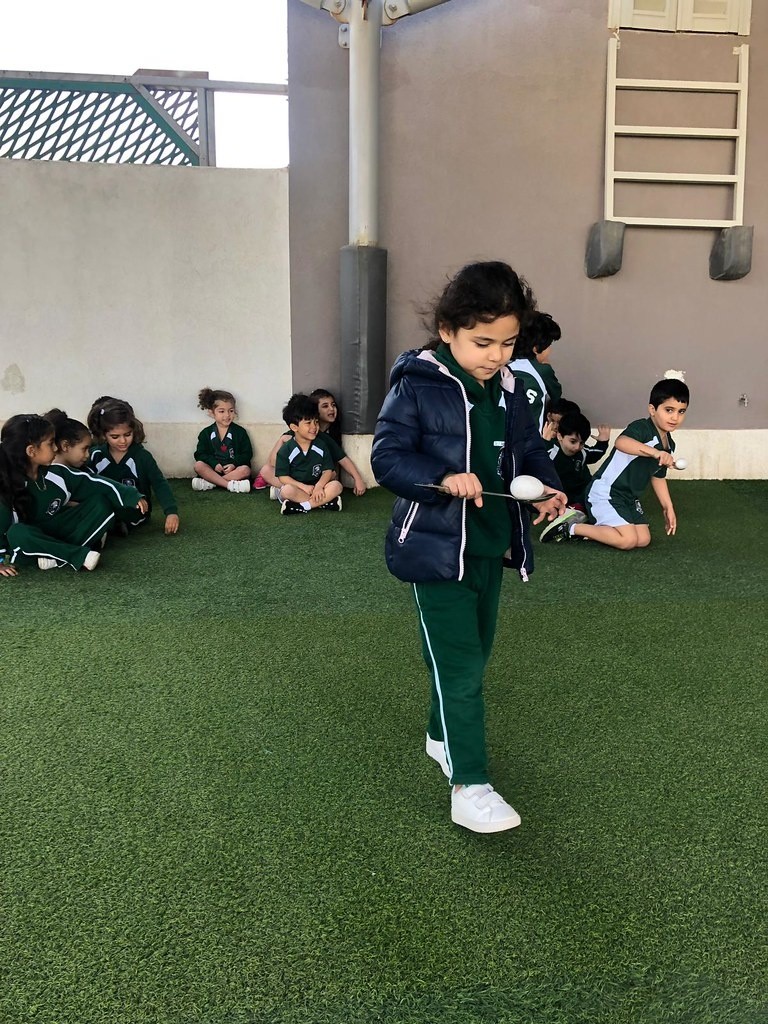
[270,484,281,500]
[566,502,587,521]
[253,471,271,489]
[95,531,108,548]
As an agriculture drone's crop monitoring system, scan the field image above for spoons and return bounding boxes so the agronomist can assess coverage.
[413,483,558,502]
[639,450,686,470]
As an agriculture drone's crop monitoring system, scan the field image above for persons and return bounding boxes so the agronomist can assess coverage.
[192,388,253,494]
[496,308,610,543]
[370,261,568,835]
[252,388,367,515]
[538,379,690,550]
[0,395,180,578]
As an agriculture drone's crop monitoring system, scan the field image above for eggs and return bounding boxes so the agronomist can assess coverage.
[510,474,544,500]
[675,458,688,469]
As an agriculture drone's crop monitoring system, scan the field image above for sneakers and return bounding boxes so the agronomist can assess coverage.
[280,499,308,514]
[38,557,57,570]
[426,732,453,779]
[82,550,101,571]
[192,477,216,491]
[540,509,578,543]
[227,479,251,493]
[310,494,342,512]
[450,783,521,834]
